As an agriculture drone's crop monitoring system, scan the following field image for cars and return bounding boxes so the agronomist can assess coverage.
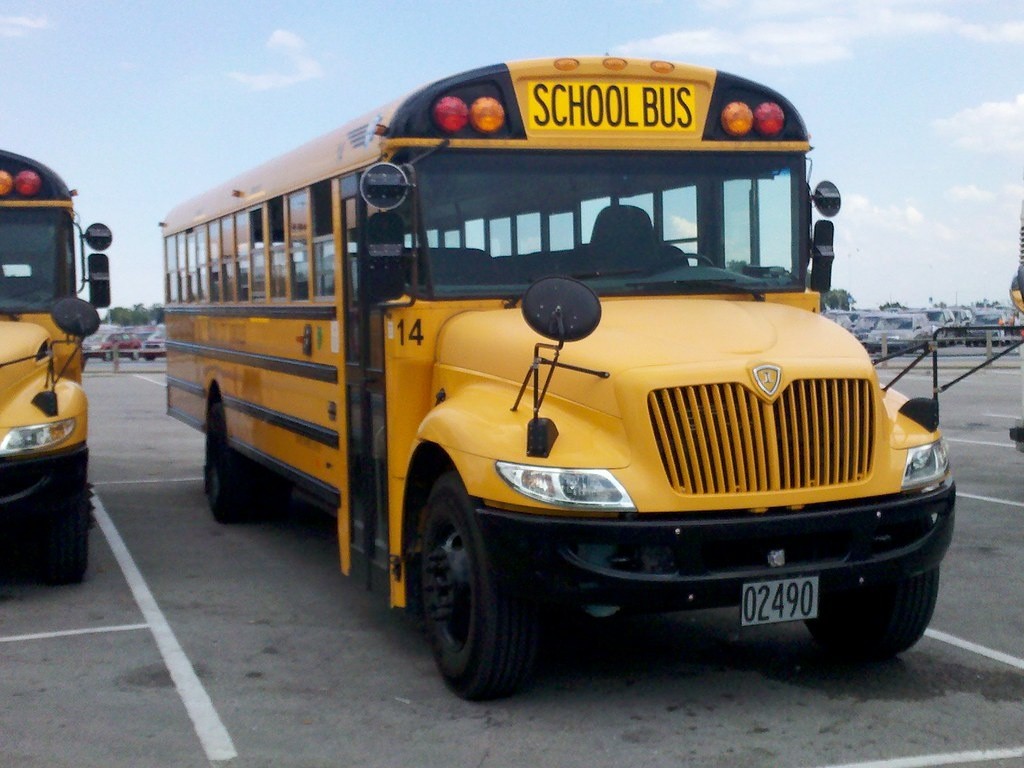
[81,322,165,361]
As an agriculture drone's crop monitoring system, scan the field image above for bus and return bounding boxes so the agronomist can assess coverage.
[1,149,112,587]
[159,53,959,704]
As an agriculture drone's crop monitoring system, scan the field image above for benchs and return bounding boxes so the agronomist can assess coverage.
[406,243,690,286]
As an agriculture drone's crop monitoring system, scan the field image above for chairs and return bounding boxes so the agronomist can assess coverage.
[585,204,661,278]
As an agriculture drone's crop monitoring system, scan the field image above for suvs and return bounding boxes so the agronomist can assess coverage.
[823,303,1024,354]
